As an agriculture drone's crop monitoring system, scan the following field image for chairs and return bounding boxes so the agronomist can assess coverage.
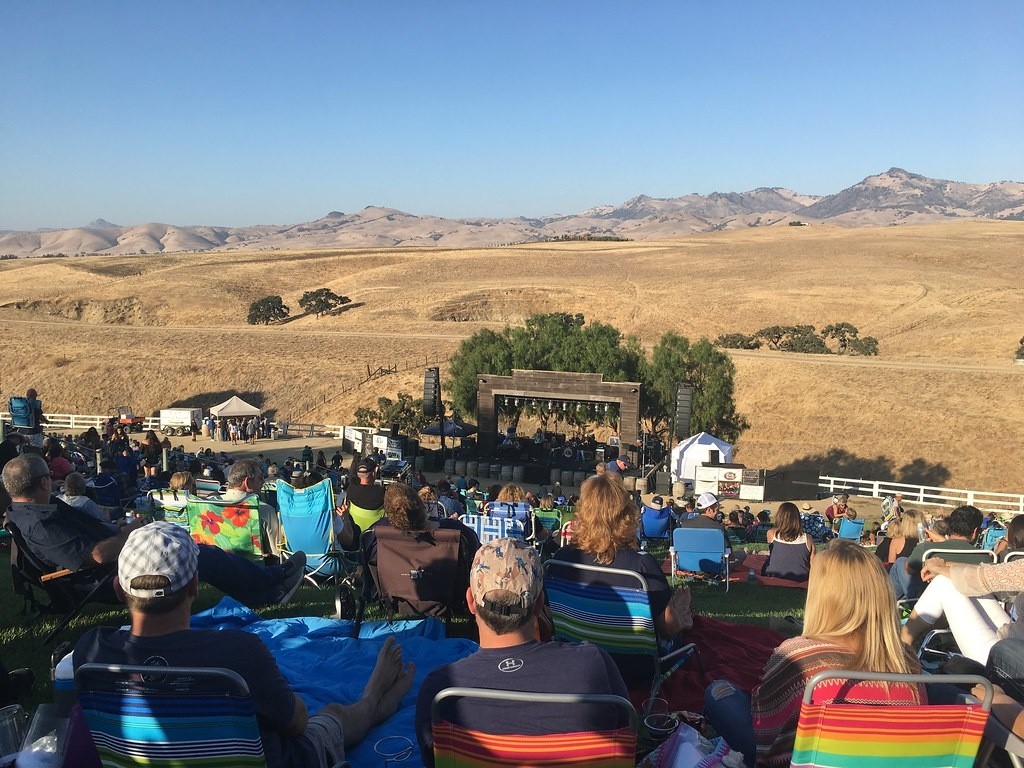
[1,477,1024,768]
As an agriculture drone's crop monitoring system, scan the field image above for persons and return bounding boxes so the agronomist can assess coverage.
[0,416,1024,768]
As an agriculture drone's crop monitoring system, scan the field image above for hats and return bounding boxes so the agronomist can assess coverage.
[5,432,25,445]
[470,537,543,617]
[437,480,451,491]
[652,496,663,505]
[696,492,724,509]
[617,455,632,467]
[88,427,98,436]
[117,519,200,598]
[356,458,379,473]
[895,493,903,496]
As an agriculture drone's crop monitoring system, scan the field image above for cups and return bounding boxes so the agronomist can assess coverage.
[642,698,676,748]
[0,705,33,768]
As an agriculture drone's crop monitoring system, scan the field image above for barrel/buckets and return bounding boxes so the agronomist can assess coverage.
[134,496,149,510]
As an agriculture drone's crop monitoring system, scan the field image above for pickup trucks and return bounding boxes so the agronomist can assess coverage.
[101,406,145,434]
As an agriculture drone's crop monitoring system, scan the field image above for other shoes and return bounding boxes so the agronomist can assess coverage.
[271,551,307,584]
[270,565,305,607]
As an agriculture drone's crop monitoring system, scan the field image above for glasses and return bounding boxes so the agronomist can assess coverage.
[620,462,627,466]
[248,474,264,484]
[38,470,54,483]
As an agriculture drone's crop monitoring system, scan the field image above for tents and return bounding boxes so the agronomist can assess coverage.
[670,431,732,483]
[421,420,478,436]
[210,396,261,441]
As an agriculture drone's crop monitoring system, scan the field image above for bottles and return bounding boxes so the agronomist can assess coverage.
[126,512,132,523]
[748,569,756,583]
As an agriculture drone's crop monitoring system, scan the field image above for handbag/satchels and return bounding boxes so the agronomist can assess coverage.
[881,495,898,523]
